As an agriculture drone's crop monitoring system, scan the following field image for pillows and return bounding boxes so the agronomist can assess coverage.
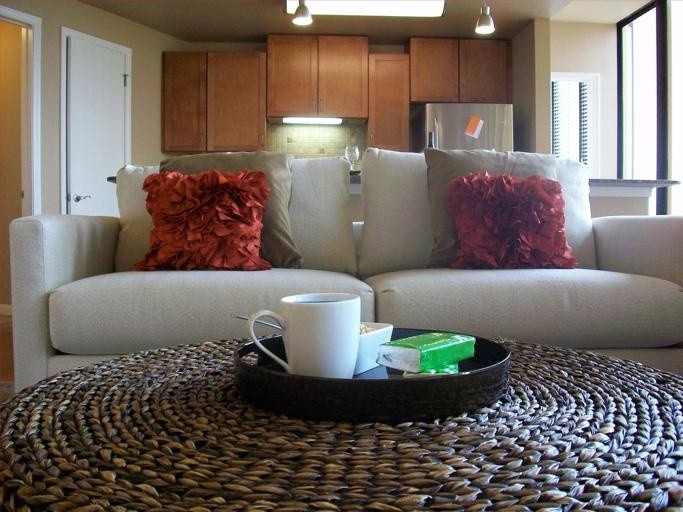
[357,147,597,275]
[134,172,274,271]
[425,146,559,269]
[159,151,302,269]
[116,157,356,277]
[449,174,577,269]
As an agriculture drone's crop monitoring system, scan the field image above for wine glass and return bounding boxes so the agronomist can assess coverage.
[346,145,361,174]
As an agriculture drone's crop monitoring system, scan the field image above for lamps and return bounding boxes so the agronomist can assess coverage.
[292,0,314,29]
[474,1,495,37]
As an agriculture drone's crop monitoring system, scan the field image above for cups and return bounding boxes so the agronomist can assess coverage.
[248,292,361,379]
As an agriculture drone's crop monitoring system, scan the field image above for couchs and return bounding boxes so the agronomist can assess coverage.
[10,146,675,393]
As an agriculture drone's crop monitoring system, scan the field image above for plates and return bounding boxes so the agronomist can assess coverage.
[234,328,511,421]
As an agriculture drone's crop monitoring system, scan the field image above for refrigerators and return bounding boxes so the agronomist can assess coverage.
[416,102,515,151]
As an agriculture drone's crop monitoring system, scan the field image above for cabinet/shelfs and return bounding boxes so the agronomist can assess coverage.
[266,32,369,119]
[368,53,411,152]
[410,36,513,103]
[162,50,266,153]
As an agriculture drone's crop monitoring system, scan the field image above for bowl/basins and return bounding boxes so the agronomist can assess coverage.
[354,322,393,376]
